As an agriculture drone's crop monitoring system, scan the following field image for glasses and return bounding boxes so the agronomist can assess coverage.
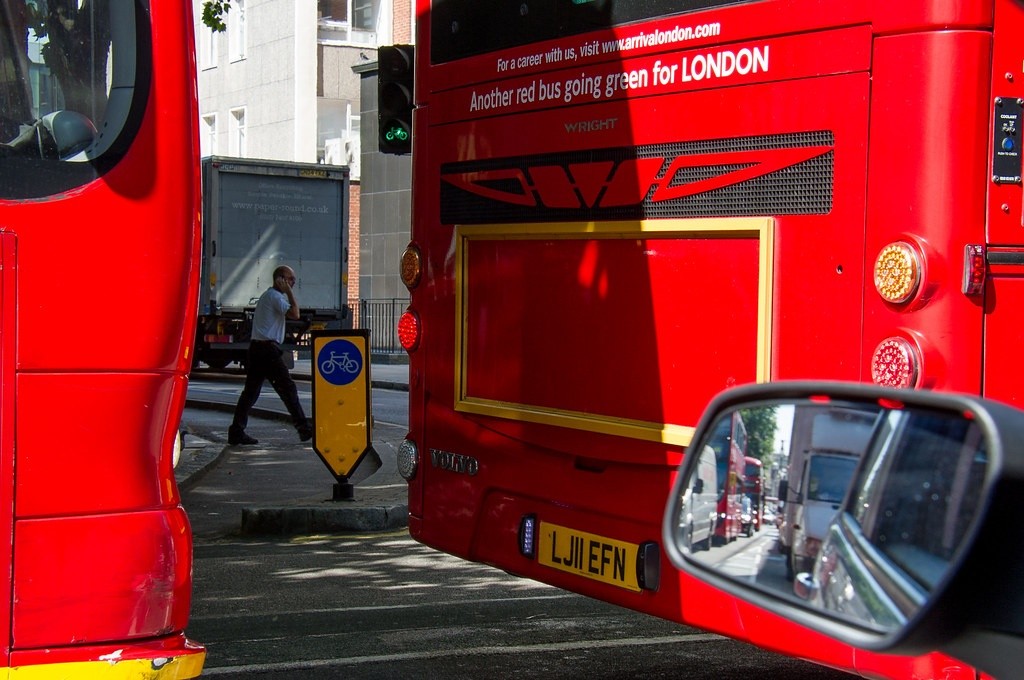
[281,276,296,281]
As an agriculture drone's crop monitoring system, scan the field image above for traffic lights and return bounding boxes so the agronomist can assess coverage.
[376,45,413,159]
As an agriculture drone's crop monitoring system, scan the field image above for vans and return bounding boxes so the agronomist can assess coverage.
[676,445,721,555]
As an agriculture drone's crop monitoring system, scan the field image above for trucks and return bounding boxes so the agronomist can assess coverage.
[778,403,882,582]
[195,155,355,371]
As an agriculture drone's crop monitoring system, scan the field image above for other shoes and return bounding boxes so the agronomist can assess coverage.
[228,424,258,446]
[298,423,313,442]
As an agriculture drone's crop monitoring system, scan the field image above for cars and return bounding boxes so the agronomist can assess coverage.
[764,496,778,525]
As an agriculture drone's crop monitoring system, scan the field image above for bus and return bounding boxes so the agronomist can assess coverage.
[740,455,767,532]
[398,0,1024,680]
[707,408,753,546]
[0,0,213,680]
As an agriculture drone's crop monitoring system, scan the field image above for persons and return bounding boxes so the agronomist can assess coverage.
[223,265,313,445]
